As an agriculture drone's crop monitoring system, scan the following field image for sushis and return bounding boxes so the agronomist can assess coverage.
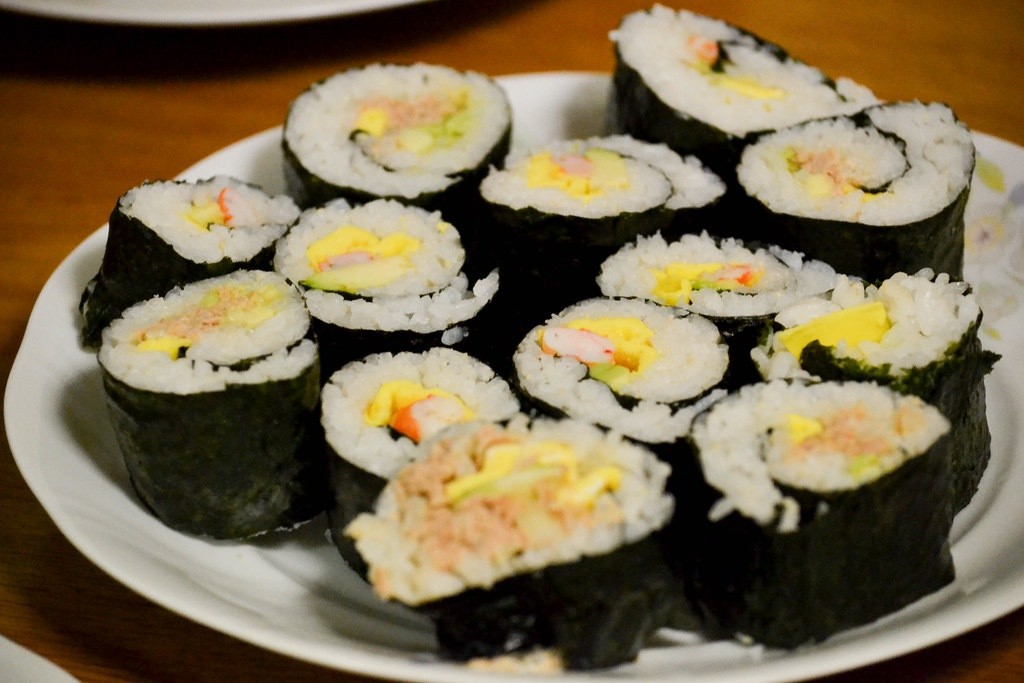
[79,0,1002,676]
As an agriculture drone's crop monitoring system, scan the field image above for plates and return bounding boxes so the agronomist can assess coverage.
[4,69,1024,682]
